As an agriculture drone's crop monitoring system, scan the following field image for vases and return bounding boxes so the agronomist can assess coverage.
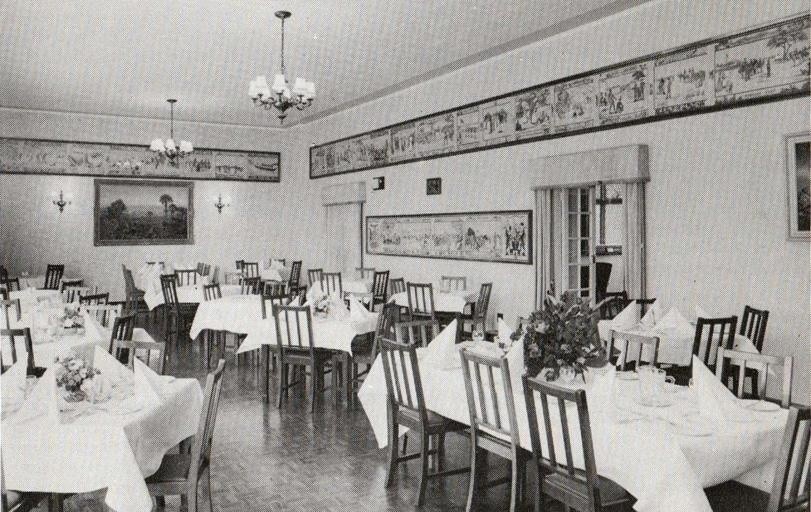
[62,385,84,403]
[313,310,328,319]
[59,323,78,334]
[547,366,575,382]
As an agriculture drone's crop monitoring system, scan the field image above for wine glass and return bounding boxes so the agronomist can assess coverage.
[472,330,484,350]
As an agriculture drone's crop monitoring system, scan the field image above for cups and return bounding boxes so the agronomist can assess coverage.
[635,365,676,406]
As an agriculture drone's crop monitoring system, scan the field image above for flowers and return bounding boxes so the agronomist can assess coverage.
[48,308,85,328]
[50,354,101,390]
[308,295,334,312]
[6,275,64,290]
[508,292,620,384]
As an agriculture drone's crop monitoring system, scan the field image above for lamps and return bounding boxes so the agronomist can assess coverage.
[50,186,71,213]
[214,192,230,214]
[149,103,193,166]
[249,14,314,126]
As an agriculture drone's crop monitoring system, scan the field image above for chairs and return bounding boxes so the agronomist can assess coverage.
[203,285,227,372]
[374,335,470,507]
[0,328,47,378]
[161,274,180,287]
[212,268,218,283]
[0,298,22,329]
[320,272,342,298]
[0,287,9,300]
[61,279,84,293]
[259,293,293,403]
[307,268,324,287]
[440,276,466,290]
[286,260,303,294]
[607,330,659,372]
[460,283,492,337]
[407,281,436,322]
[713,346,794,407]
[103,359,226,511]
[0,277,20,292]
[521,372,635,511]
[241,263,259,277]
[196,263,210,275]
[109,311,137,366]
[624,298,656,319]
[351,300,397,409]
[111,339,168,376]
[66,290,88,303]
[272,303,338,412]
[361,271,390,311]
[763,407,811,511]
[80,302,122,329]
[726,305,769,399]
[458,347,534,511]
[1,449,48,512]
[44,263,65,289]
[388,276,405,297]
[161,278,195,347]
[0,265,9,292]
[601,291,627,320]
[688,316,738,385]
[79,292,109,306]
[122,263,157,325]
[356,268,376,277]
[241,276,261,295]
[146,262,165,269]
[174,270,196,286]
[395,318,440,350]
[270,258,285,266]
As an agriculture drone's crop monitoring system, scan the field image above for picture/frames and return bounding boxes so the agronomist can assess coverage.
[367,210,533,264]
[0,136,280,182]
[426,178,441,195]
[785,131,808,237]
[309,9,811,181]
[92,176,194,244]
